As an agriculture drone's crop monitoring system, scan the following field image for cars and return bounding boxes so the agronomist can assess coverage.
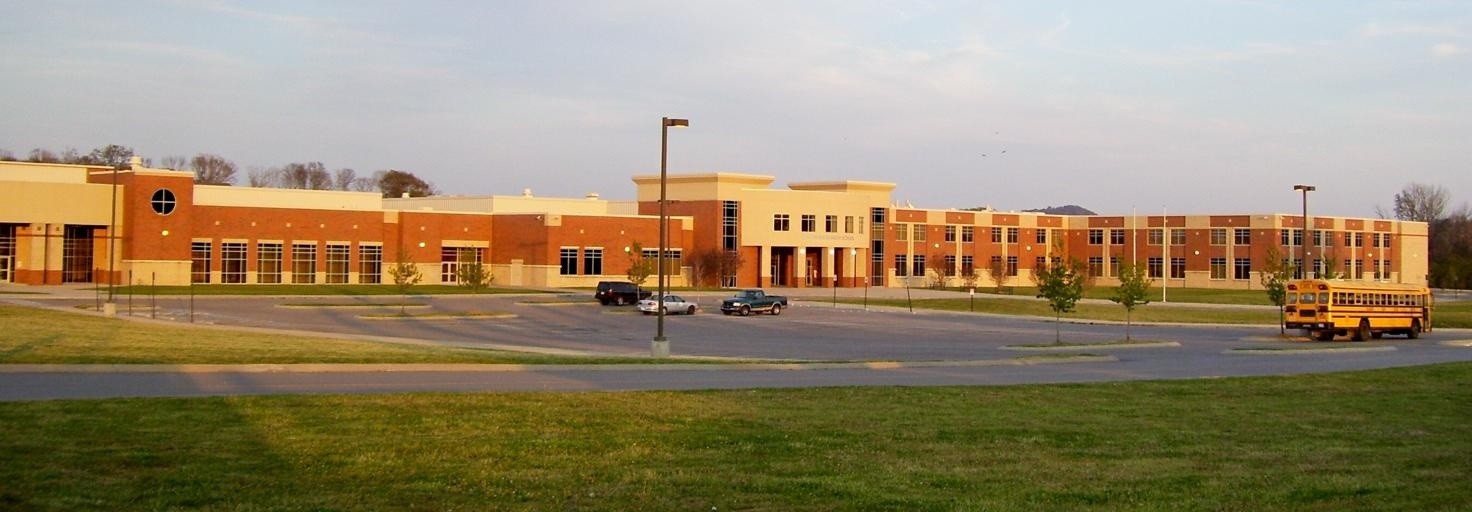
[637,294,699,314]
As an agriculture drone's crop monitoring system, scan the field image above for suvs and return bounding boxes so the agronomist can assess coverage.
[594,281,651,307]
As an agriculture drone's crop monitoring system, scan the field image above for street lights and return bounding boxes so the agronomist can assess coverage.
[658,116,688,334]
[1293,185,1316,281]
[109,162,131,301]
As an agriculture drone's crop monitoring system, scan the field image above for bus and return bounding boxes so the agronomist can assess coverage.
[1286,278,1435,342]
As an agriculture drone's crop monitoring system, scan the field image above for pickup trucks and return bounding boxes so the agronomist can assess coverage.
[721,290,787,316]
